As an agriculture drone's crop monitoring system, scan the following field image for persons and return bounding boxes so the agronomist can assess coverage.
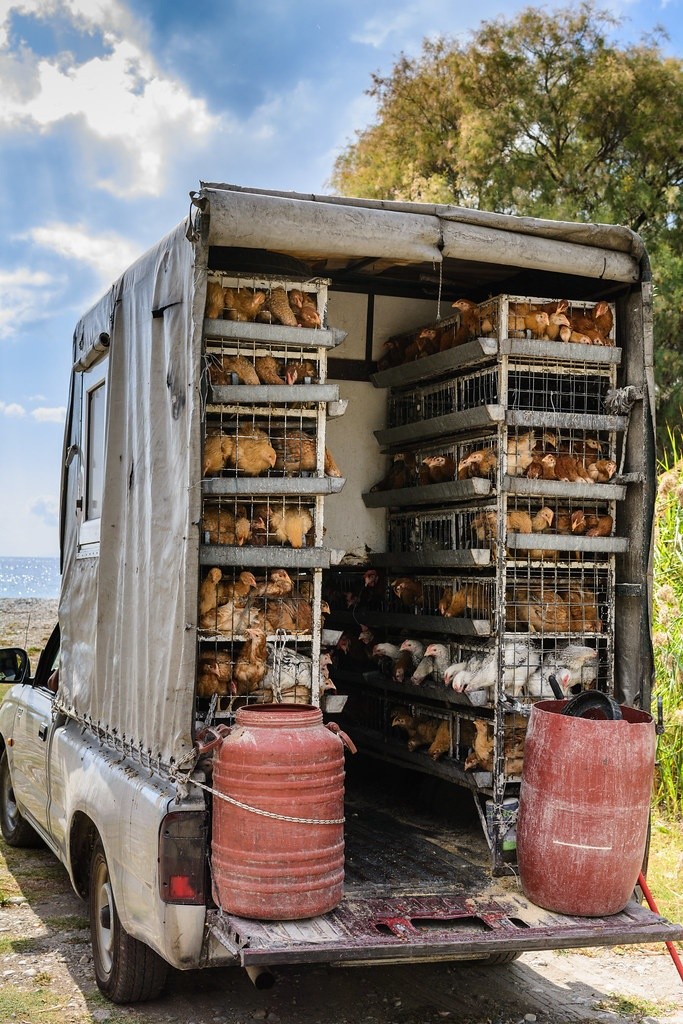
[47,646,61,693]
[0,652,22,681]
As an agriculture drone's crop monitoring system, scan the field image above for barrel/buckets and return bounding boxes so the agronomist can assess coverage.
[194,702,360,919]
[516,699,656,915]
[486,798,519,850]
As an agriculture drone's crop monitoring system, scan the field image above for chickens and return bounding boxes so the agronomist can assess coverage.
[195,283,350,712]
[359,299,614,777]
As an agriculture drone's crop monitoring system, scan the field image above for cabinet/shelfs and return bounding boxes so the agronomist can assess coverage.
[195,275,348,721]
[360,295,623,871]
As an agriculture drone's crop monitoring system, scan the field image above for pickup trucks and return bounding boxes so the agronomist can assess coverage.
[1,620,683,1006]
